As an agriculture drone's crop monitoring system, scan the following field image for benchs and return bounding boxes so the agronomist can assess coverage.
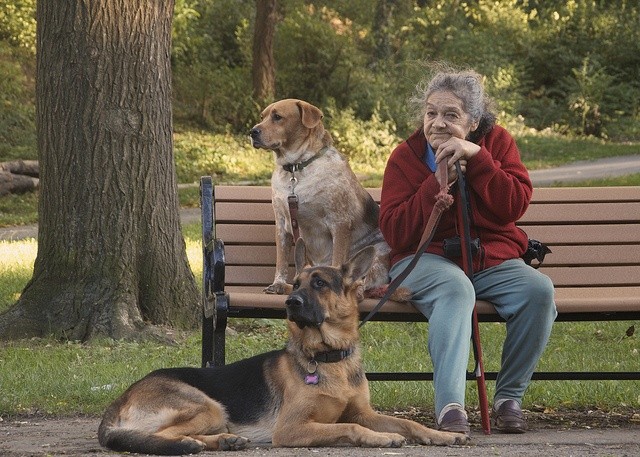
[200,172,636,383]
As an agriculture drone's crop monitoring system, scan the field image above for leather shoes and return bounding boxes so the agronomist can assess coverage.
[491,398,527,432]
[436,402,471,431]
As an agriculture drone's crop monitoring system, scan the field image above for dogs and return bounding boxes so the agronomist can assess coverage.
[97,237,472,456]
[248,98,413,304]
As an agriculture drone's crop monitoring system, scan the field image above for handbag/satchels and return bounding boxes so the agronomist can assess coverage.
[519,239,551,268]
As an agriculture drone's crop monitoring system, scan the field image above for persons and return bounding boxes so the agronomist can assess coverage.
[378,60,558,433]
